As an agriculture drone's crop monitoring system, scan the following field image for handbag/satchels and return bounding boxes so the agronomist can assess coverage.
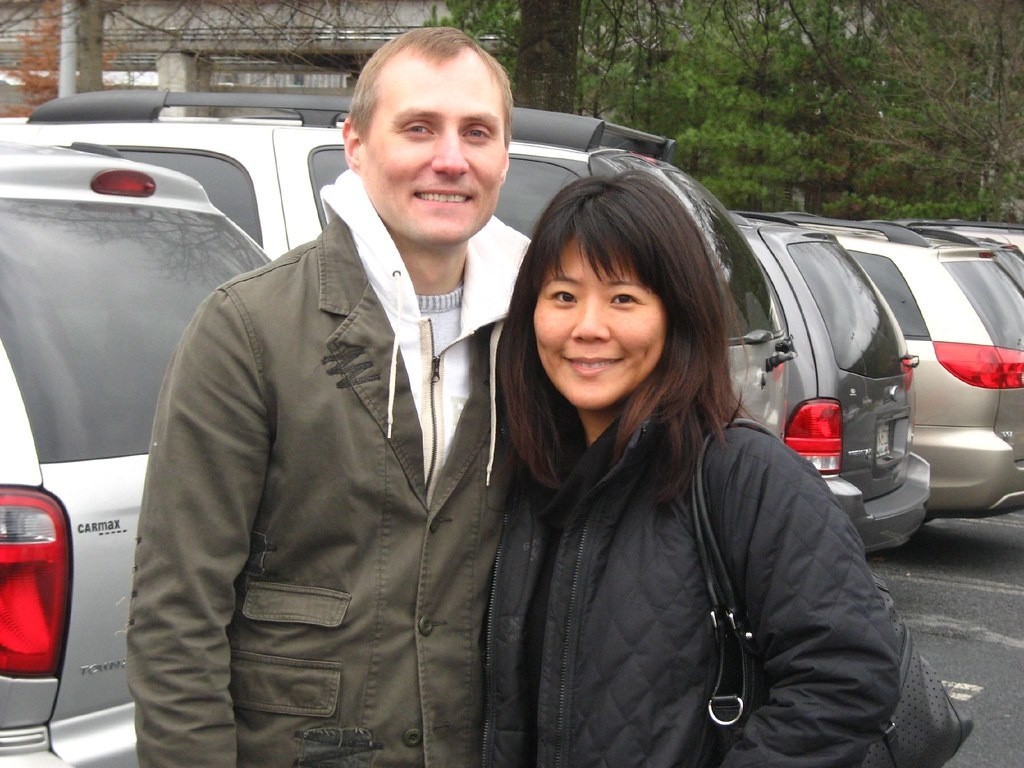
[690,417,975,768]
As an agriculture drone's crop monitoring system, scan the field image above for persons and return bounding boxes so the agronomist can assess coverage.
[126,24,534,768]
[478,167,899,767]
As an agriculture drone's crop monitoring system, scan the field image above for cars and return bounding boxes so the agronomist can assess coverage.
[0,137,300,767]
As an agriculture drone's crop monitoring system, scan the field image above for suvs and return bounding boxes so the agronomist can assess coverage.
[0,86,795,456]
[741,209,1024,518]
[724,208,933,561]
